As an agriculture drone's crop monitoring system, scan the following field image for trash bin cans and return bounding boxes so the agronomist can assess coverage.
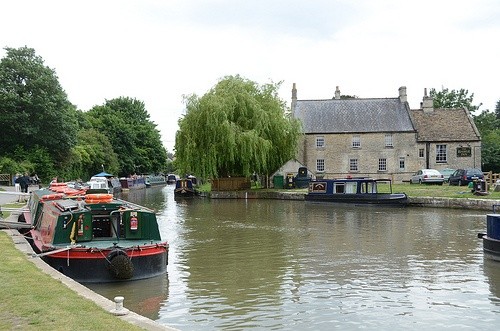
[273,175,284,189]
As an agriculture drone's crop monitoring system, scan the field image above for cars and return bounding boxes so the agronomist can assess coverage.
[449,167,484,187]
[409,169,445,185]
[439,168,455,183]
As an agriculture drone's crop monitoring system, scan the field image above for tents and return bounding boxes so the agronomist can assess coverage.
[95,172,114,177]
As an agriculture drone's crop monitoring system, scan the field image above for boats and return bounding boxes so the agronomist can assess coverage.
[303,176,408,204]
[17,175,168,286]
[63,165,198,204]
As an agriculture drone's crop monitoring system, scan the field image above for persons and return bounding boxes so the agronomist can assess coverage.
[14,172,29,202]
